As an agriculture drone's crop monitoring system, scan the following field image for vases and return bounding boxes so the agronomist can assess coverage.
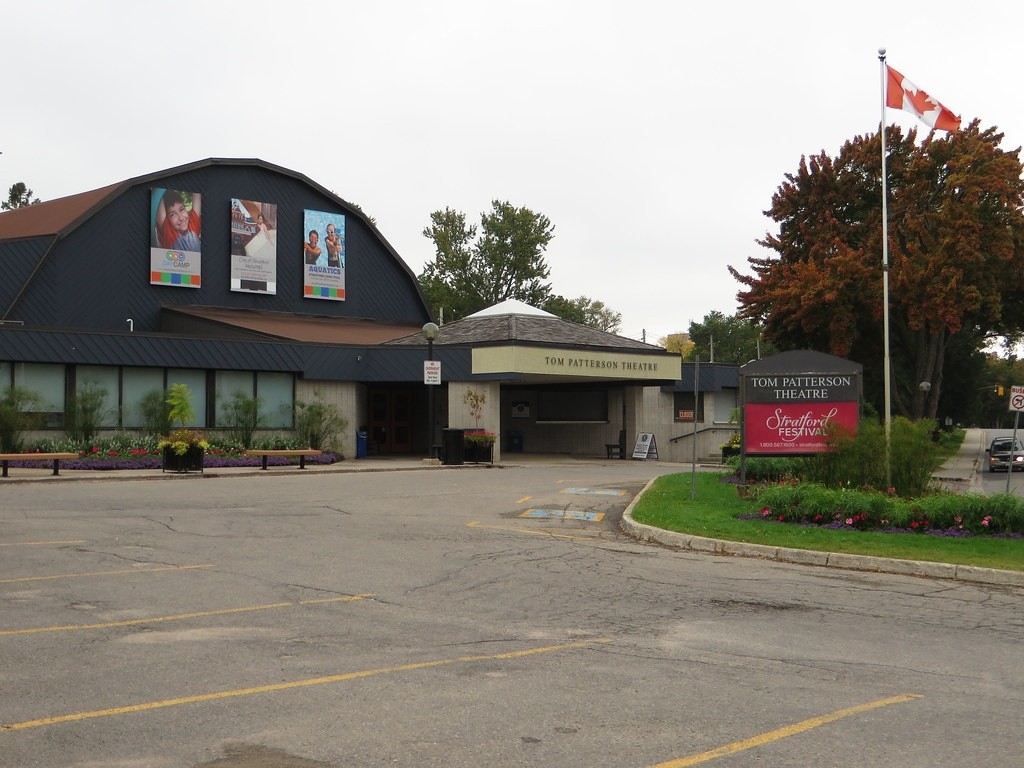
[162,444,205,473]
[465,447,493,464]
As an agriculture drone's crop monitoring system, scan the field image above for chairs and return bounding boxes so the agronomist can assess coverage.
[605,431,626,460]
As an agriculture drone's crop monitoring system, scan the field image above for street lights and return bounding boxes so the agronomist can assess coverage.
[423,323,441,466]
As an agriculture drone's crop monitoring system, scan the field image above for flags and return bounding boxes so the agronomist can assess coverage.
[886,63,961,133]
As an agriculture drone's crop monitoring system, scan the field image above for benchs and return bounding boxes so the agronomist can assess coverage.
[246,448,321,469]
[0,452,80,477]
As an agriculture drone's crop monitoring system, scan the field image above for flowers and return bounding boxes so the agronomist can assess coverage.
[156,429,211,455]
[466,429,495,444]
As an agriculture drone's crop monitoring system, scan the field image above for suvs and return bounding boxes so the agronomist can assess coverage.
[986,437,1024,472]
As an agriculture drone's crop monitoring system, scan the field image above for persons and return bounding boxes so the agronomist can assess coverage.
[244,215,270,240]
[379,398,386,440]
[155,189,201,252]
[304,230,322,265]
[325,224,342,268]
